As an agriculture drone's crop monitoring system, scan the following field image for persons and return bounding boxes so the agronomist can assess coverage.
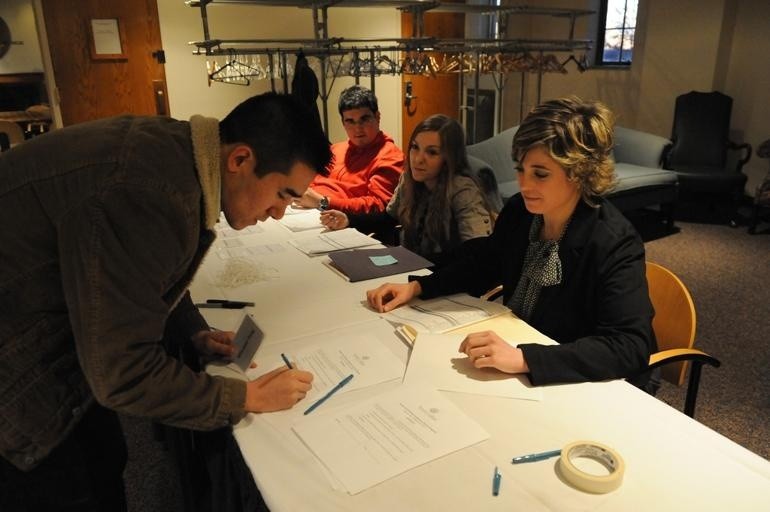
[320,114,494,262]
[291,85,407,218]
[0,92,333,511]
[365,94,663,400]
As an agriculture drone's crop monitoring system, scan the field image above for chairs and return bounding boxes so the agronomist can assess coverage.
[662,91,752,227]
[748,138,770,234]
[646,259,721,416]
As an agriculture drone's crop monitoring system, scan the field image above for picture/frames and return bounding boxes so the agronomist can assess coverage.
[84,15,129,61]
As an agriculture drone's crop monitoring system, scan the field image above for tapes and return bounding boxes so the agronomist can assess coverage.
[560,440,625,494]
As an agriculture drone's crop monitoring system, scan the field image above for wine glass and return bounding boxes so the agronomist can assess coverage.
[225,54,294,80]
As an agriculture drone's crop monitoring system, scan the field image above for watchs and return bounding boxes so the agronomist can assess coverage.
[320,194,328,211]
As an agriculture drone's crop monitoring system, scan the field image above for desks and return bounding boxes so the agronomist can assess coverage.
[187,294,770,512]
[187,202,467,350]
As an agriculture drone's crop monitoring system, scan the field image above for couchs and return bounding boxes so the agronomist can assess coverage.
[462,124,680,233]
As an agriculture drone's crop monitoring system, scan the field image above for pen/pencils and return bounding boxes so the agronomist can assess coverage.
[304,374,353,415]
[206,300,254,306]
[513,450,561,463]
[281,353,293,369]
[320,205,329,210]
[196,302,245,308]
